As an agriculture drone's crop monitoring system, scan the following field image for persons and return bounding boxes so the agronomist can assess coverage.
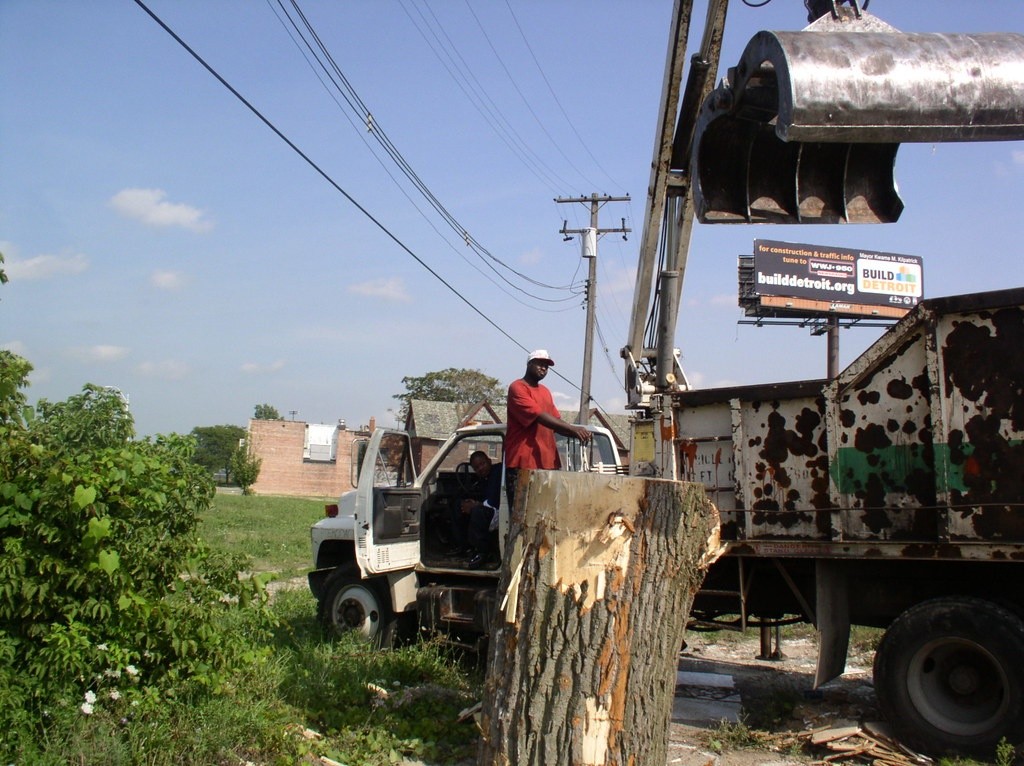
[504,350,591,512]
[438,451,500,570]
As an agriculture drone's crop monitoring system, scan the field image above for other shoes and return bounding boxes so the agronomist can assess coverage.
[463,546,486,568]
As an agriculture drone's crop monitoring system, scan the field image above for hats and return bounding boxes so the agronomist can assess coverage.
[527,350,554,366]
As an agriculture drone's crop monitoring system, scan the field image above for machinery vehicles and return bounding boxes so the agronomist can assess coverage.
[301,0,1023,759]
